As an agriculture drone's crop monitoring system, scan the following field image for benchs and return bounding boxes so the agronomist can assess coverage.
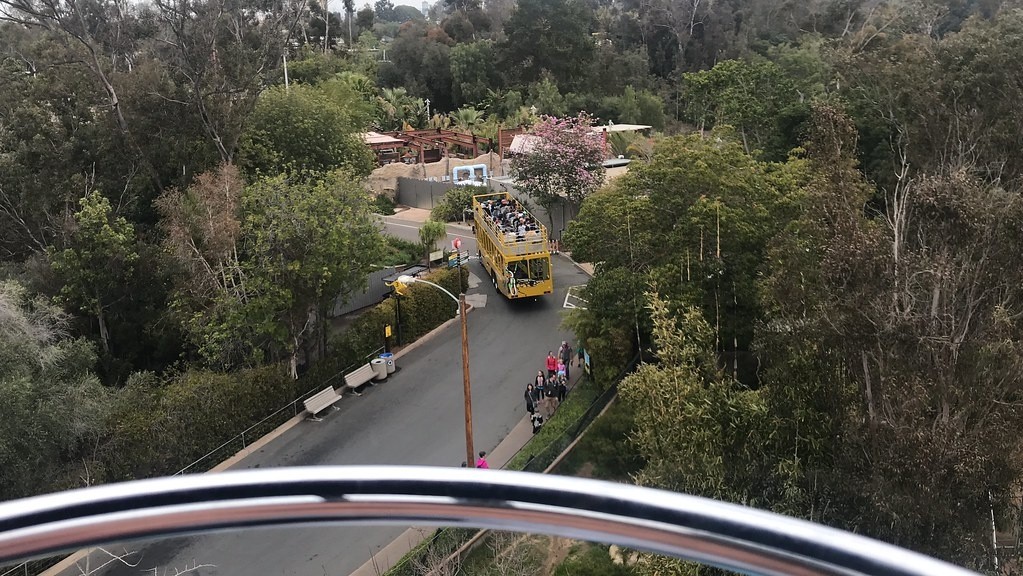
[481,199,538,236]
[304,385,343,421]
[344,363,380,396]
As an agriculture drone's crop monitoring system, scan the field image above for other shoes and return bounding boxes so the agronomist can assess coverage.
[546,416,550,419]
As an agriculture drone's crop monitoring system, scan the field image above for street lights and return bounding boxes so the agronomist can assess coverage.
[398,274,474,468]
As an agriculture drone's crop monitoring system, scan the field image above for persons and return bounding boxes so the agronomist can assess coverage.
[477,451,489,469]
[546,351,567,403]
[531,408,543,434]
[477,197,539,242]
[576,340,585,368]
[543,374,559,420]
[534,370,546,403]
[524,384,537,422]
[557,341,573,380]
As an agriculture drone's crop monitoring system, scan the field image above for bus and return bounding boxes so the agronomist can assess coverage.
[472,192,553,302]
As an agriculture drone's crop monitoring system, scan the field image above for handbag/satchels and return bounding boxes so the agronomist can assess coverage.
[532,402,537,409]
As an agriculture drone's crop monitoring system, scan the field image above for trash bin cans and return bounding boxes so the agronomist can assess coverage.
[371,358,387,380]
[379,353,396,374]
